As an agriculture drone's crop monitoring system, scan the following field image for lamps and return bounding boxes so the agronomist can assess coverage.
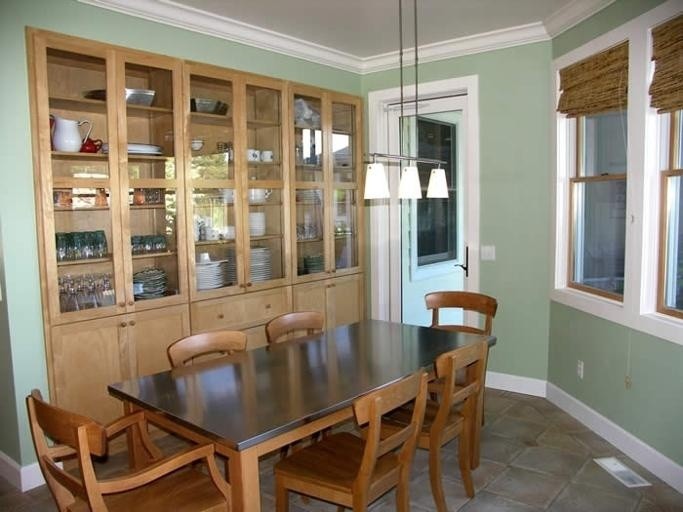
[363,1,449,200]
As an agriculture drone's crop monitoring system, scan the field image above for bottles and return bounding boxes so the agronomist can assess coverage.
[296,145,303,166]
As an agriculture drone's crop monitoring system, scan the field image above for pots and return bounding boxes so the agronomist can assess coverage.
[247,188,272,206]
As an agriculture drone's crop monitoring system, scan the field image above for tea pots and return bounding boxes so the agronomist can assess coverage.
[49,112,91,153]
[82,135,102,153]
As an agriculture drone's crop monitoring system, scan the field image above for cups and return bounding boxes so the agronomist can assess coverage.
[57,231,108,262]
[248,149,273,162]
[133,234,168,253]
[130,189,169,206]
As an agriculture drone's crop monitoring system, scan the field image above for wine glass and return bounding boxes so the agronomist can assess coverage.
[61,271,114,311]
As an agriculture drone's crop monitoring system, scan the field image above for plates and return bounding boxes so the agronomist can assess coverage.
[202,224,237,243]
[126,143,162,157]
[134,269,169,299]
[250,213,265,237]
[197,262,226,290]
[228,246,272,282]
[303,255,326,272]
[295,189,322,202]
